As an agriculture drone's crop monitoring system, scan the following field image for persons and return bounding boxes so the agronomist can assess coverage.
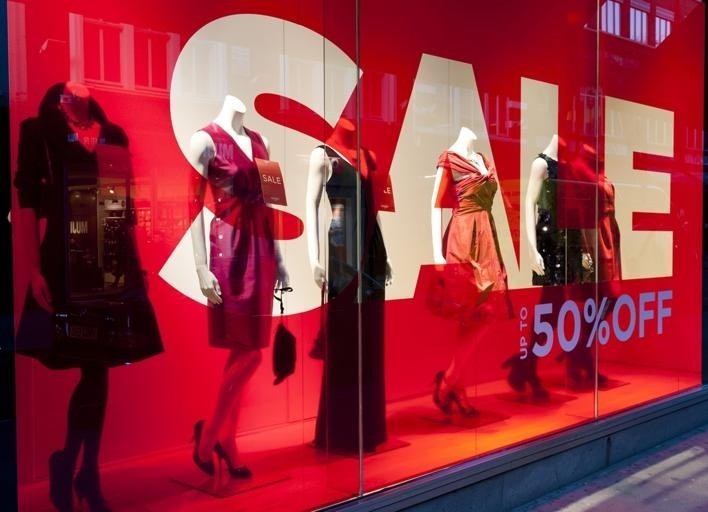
[557,144,624,386]
[499,133,591,400]
[430,124,517,416]
[188,94,290,480]
[14,81,166,511]
[306,117,395,456]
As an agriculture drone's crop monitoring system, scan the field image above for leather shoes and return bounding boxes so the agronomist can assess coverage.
[48,449,74,512]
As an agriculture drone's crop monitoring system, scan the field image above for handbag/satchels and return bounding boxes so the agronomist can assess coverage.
[272,286,297,385]
[307,282,329,361]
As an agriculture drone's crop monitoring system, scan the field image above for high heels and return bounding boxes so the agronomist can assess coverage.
[501,354,549,400]
[187,419,216,476]
[214,440,253,479]
[556,342,608,385]
[75,469,111,512]
[449,384,480,417]
[431,370,452,416]
